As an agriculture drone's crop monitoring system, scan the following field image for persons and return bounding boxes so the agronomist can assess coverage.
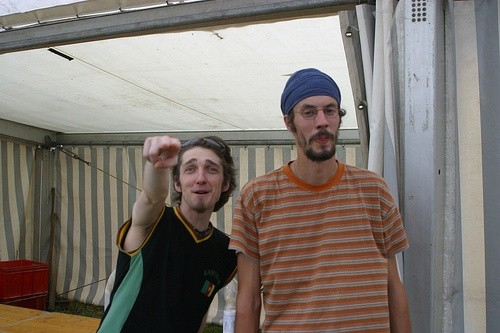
[92,133,241,333]
[229,68,415,333]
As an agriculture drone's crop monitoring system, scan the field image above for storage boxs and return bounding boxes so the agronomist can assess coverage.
[1,257,48,313]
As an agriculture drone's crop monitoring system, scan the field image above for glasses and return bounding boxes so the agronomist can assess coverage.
[293,107,339,119]
[180,138,223,149]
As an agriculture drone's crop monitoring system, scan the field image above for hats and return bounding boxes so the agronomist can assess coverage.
[280,68,341,113]
